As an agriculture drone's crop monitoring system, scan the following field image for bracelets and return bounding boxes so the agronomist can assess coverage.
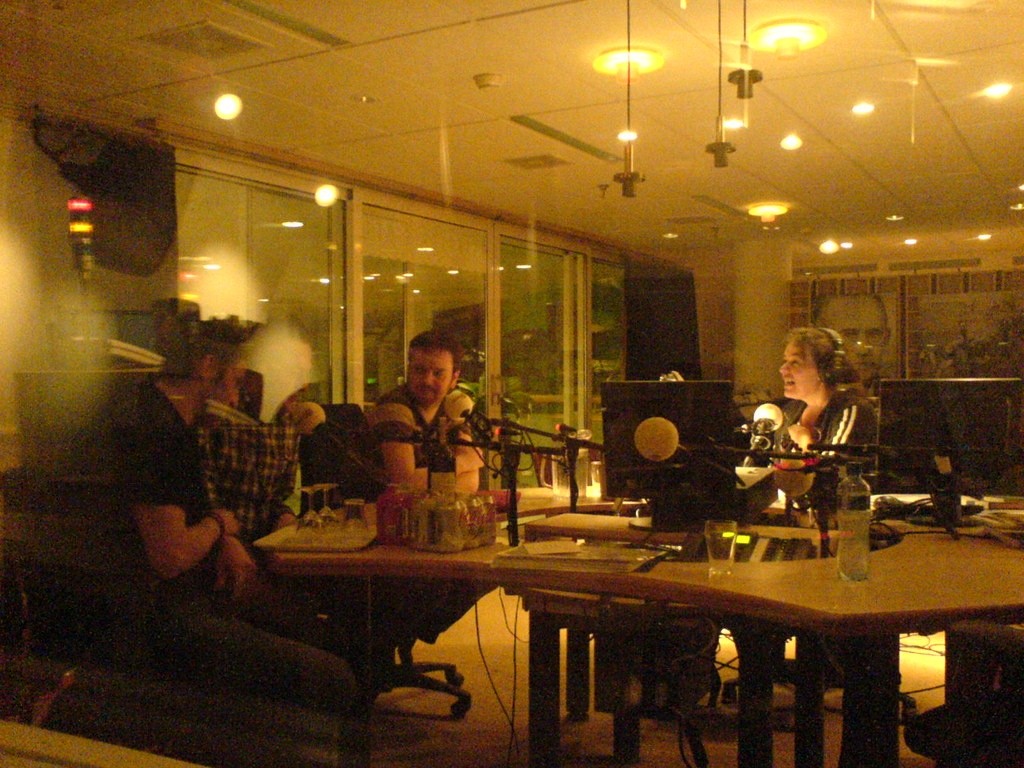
[204,512,225,539]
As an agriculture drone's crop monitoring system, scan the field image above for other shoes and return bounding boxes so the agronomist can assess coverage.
[721,673,741,705]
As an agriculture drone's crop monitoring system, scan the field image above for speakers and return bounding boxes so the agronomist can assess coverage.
[92,132,175,279]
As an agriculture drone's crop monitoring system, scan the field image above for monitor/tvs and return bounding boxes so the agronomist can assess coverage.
[600,378,735,533]
[875,377,1024,528]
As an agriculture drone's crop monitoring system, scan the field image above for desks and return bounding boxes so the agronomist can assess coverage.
[252,486,1024,768]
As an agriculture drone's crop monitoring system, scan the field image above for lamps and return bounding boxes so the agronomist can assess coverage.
[747,202,790,226]
[589,44,670,83]
[744,14,831,61]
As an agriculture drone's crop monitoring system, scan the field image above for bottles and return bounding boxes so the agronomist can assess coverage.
[834,459,872,582]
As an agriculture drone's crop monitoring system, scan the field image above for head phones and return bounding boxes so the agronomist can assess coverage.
[818,327,848,385]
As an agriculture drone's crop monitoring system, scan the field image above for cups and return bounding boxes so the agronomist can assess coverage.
[339,499,370,541]
[705,520,737,576]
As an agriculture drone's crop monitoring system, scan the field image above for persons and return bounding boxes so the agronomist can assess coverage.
[657,326,873,513]
[82,304,362,704]
[367,330,491,668]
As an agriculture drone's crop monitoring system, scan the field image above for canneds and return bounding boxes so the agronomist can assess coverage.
[373,490,497,552]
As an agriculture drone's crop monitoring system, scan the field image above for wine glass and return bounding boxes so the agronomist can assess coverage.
[313,484,340,540]
[296,483,326,547]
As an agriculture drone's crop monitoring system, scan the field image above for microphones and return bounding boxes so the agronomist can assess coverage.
[752,403,785,442]
[292,403,369,471]
[443,390,491,443]
[633,416,734,480]
